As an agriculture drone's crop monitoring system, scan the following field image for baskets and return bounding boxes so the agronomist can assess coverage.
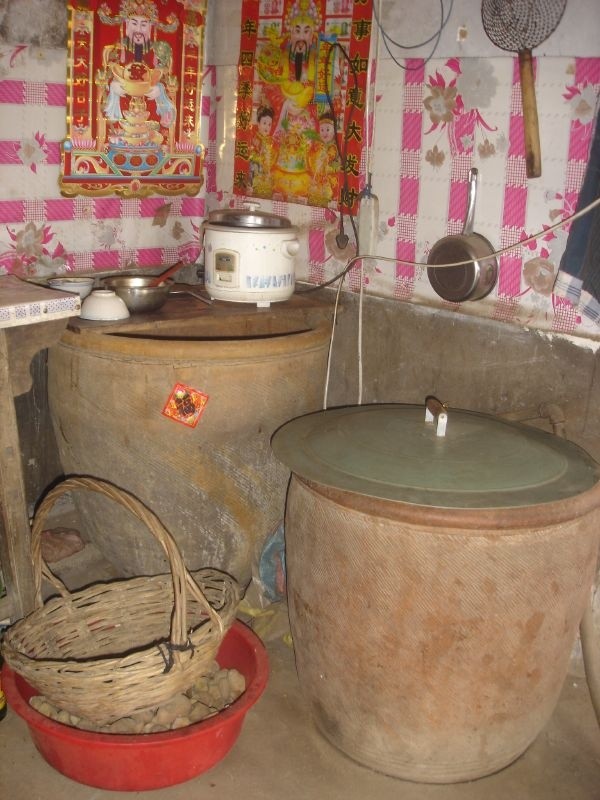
[0,477,241,725]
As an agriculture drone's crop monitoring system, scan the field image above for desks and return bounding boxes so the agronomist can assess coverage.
[0,274,81,625]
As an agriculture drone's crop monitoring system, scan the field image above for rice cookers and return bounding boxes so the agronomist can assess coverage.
[203,201,301,308]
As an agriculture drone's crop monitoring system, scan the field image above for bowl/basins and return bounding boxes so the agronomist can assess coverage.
[100,275,176,313]
[1,617,270,791]
[79,290,130,321]
[47,278,94,299]
